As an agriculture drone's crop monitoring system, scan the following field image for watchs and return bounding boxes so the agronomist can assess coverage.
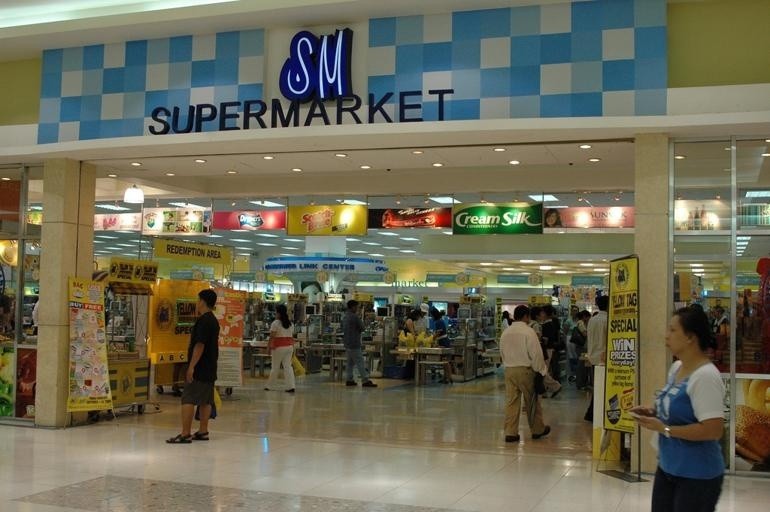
[664,425,672,439]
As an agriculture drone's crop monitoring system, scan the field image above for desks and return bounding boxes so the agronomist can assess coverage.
[243,340,455,386]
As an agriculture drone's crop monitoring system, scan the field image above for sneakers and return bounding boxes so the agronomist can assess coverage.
[345,380,358,386]
[541,387,563,398]
[531,425,552,438]
[284,387,295,393]
[503,433,522,442]
[362,380,378,387]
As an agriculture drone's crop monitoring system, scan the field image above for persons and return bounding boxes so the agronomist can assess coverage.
[340,300,377,387]
[400,302,454,384]
[166,287,220,443]
[301,281,322,303]
[501,306,562,441]
[544,209,562,228]
[709,304,728,334]
[0,294,14,334]
[264,305,295,392]
[530,306,599,398]
[501,311,512,330]
[584,295,609,421]
[629,307,724,512]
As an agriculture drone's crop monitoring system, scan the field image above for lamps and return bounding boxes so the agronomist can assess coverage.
[123,184,144,204]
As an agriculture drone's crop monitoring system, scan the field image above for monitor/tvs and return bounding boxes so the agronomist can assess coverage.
[377,307,389,316]
[305,305,316,315]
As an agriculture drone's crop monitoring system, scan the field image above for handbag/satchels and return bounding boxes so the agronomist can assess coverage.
[195,386,224,420]
[291,351,306,376]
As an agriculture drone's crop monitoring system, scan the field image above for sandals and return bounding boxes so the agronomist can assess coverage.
[191,429,211,441]
[163,432,194,444]
